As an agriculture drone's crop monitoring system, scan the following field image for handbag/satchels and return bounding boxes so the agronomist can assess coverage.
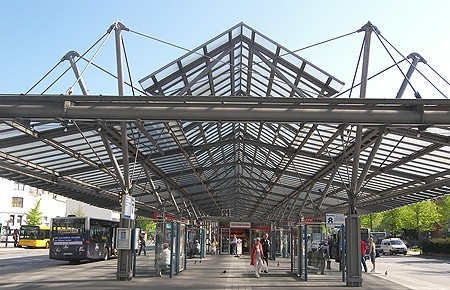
[261,260,267,270]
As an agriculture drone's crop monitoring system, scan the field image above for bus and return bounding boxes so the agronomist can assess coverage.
[17,225,75,248]
[48,214,141,266]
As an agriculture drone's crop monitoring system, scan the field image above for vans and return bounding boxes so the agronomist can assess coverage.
[380,238,408,255]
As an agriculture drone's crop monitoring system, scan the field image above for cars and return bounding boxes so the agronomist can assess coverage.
[308,239,322,252]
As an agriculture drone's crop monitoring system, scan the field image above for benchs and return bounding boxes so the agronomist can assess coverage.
[160,266,170,275]
[193,252,200,258]
[308,266,319,274]
[276,252,283,259]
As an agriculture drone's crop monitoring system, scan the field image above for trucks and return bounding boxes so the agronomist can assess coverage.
[370,231,388,251]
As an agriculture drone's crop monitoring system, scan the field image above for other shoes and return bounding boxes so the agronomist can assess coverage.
[371,269,375,272]
[254,275,261,277]
[264,270,268,273]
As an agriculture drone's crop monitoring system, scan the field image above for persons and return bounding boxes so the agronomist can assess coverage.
[137,234,147,256]
[305,233,377,275]
[156,242,171,277]
[191,232,271,278]
[12,231,18,247]
